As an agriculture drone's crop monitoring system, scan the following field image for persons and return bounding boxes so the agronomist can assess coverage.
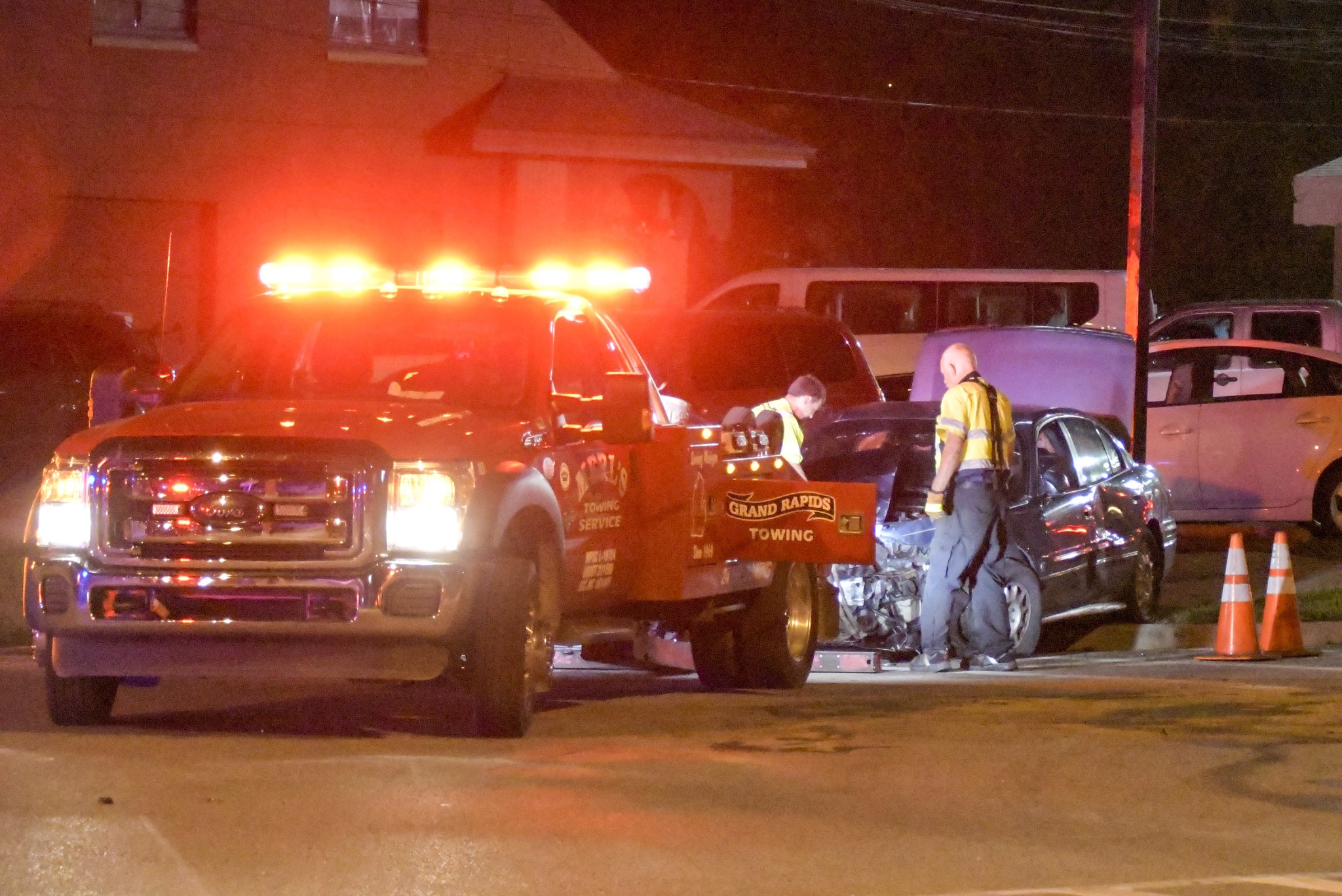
[909,340,1017,671]
[750,373,828,482]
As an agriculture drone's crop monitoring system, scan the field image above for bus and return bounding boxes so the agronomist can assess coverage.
[689,264,1126,404]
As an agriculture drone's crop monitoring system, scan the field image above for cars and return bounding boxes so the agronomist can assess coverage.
[1148,341,1341,536]
[800,397,1175,655]
[610,315,886,460]
[1152,298,1341,355]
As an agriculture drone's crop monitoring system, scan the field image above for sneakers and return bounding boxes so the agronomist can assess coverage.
[910,651,951,673]
[965,649,1018,671]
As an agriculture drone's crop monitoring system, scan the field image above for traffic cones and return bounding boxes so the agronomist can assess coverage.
[1192,533,1282,662]
[1264,532,1324,657]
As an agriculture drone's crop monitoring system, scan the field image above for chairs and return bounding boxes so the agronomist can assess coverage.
[1279,354,1333,397]
[1186,352,1218,403]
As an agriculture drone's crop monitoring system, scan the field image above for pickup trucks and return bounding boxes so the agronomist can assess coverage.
[24,262,880,740]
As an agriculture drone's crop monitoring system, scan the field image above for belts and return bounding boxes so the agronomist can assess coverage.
[955,481,1008,490]
